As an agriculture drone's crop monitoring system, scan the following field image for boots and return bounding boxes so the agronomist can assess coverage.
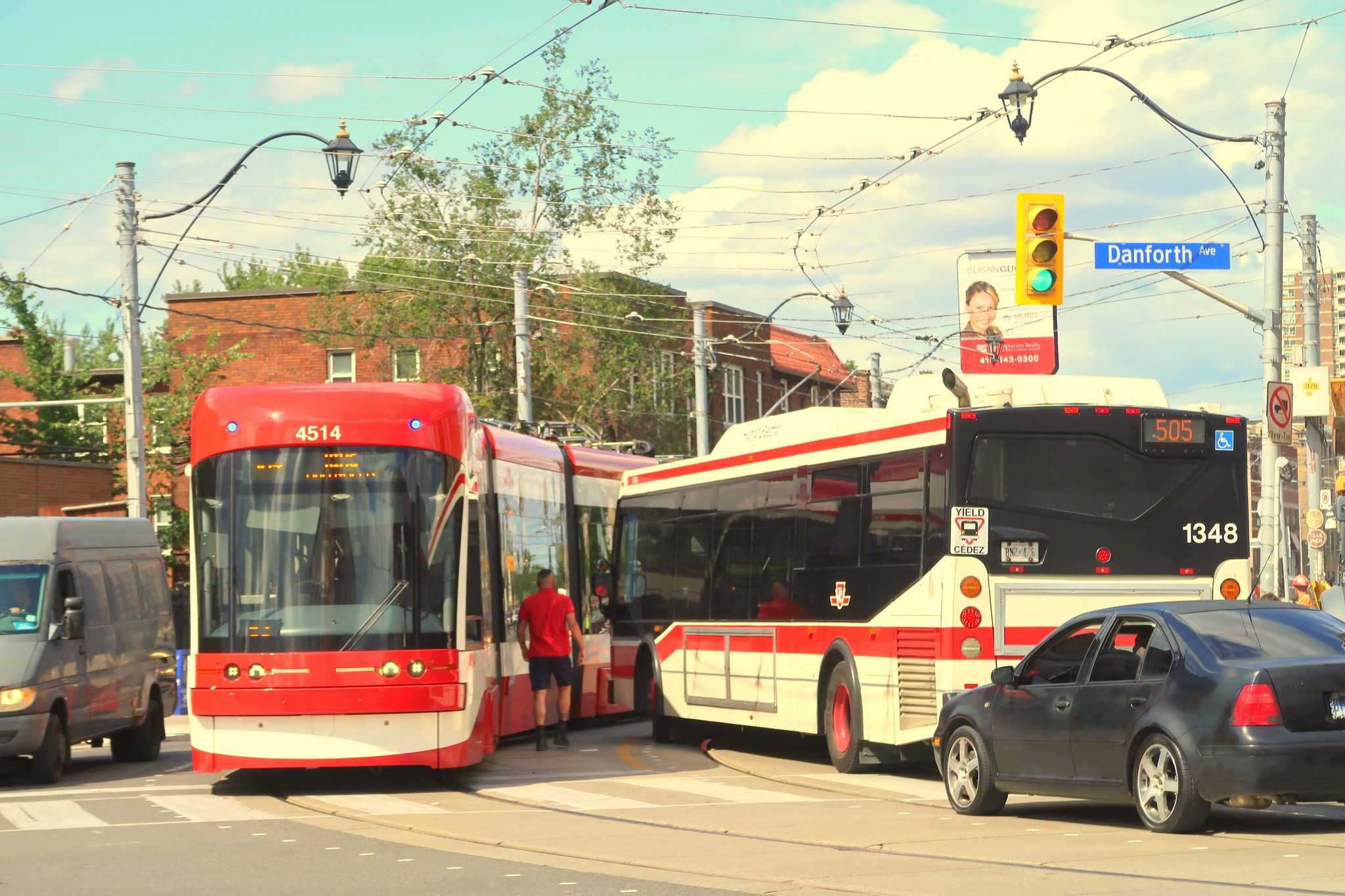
[536,731,548,752]
[553,724,569,746]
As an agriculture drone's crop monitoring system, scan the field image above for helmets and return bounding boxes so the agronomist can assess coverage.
[1291,575,1310,587]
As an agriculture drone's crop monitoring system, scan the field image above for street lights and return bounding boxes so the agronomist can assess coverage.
[691,286,857,458]
[868,328,1006,408]
[112,115,365,517]
[998,59,1290,600]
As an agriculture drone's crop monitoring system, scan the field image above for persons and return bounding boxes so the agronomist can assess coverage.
[516,569,586,752]
[1292,574,1313,608]
[757,581,807,620]
[1260,593,1280,601]
[1132,630,1152,653]
[1,580,38,623]
[962,281,1002,339]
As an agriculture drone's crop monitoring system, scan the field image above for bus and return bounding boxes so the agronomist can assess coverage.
[181,382,696,776]
[602,364,1262,777]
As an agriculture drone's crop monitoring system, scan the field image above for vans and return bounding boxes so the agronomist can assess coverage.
[0,515,180,784]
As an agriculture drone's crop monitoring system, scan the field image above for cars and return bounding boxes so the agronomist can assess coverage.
[932,594,1345,836]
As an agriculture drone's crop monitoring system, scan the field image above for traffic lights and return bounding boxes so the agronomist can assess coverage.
[1015,191,1064,306]
